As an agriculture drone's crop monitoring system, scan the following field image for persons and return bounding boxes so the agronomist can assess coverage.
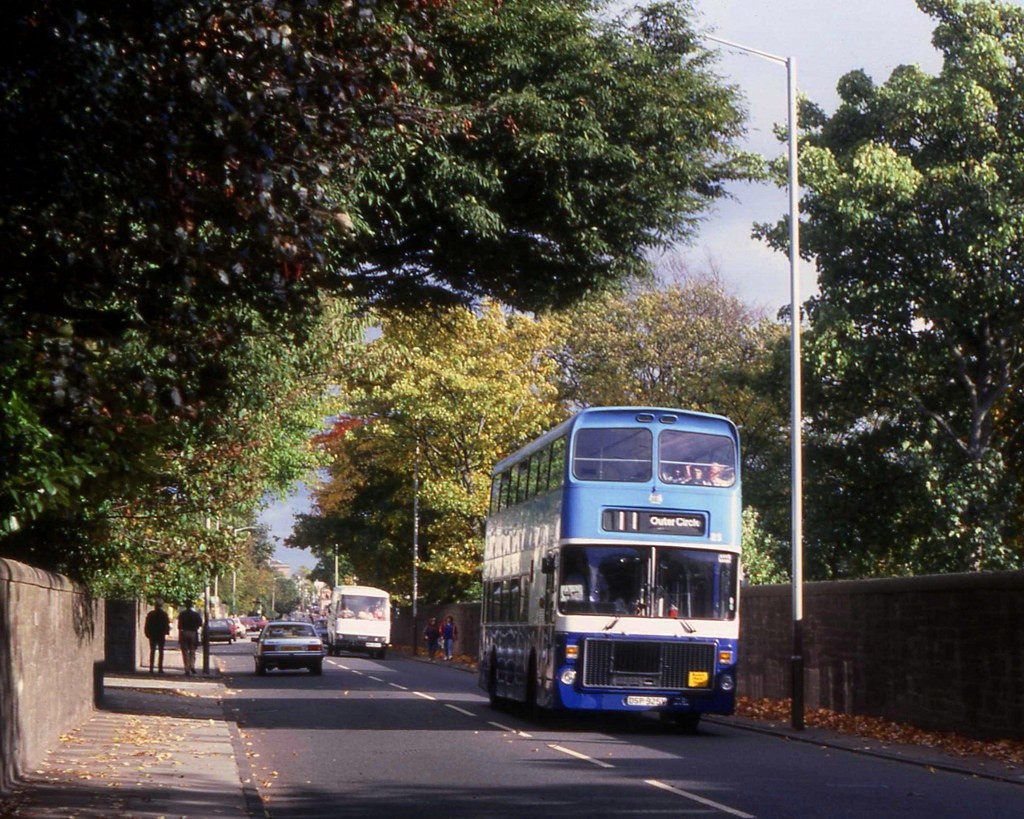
[358,605,372,617]
[667,462,725,485]
[422,616,441,662]
[340,604,354,614]
[145,598,171,673]
[178,599,202,676]
[439,616,458,660]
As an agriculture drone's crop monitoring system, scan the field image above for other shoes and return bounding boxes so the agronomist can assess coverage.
[186,673,190,677]
[191,668,196,673]
[444,655,453,661]
[159,670,163,672]
[427,658,436,663]
[149,670,153,674]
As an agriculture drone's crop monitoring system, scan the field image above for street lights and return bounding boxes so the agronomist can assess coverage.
[230,526,257,611]
[367,410,420,656]
[645,15,804,728]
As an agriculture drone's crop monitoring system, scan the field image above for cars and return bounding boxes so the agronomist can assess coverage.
[208,615,260,645]
[250,620,328,675]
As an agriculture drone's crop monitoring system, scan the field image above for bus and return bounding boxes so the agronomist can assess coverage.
[479,405,744,733]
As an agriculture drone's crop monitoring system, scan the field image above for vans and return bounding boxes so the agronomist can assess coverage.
[326,584,392,660]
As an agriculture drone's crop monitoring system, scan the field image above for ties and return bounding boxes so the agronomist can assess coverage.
[582,572,590,601]
